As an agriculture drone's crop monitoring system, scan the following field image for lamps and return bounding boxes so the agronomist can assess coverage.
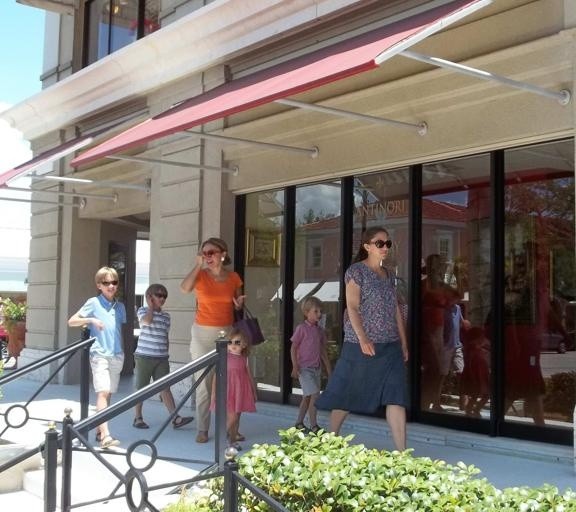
[103,2,120,15]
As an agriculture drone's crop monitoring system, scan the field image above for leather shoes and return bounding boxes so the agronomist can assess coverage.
[227,432,245,441]
[196,436,206,443]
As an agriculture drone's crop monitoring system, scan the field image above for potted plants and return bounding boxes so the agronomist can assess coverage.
[0,296,27,367]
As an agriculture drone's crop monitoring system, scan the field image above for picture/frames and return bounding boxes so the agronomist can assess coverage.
[245,227,282,268]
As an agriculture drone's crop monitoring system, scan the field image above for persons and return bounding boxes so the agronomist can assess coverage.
[419,247,563,426]
[290,295,334,437]
[133,283,195,430]
[68,266,127,450]
[210,327,258,452]
[180,237,247,444]
[313,226,410,453]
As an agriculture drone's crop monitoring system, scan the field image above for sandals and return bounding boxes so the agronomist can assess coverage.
[95,432,100,442]
[295,423,310,434]
[172,416,194,429]
[100,435,120,449]
[310,425,326,435]
[133,417,148,429]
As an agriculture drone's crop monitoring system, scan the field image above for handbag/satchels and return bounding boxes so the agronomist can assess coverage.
[232,304,265,346]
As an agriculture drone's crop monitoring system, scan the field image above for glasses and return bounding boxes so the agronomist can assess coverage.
[228,340,241,345]
[200,250,221,258]
[154,293,167,299]
[97,281,118,286]
[370,240,392,248]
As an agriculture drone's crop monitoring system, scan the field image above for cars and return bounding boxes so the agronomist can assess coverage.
[0,316,11,362]
[507,322,568,354]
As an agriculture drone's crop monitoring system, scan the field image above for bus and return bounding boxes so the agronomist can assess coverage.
[565,301,576,351]
